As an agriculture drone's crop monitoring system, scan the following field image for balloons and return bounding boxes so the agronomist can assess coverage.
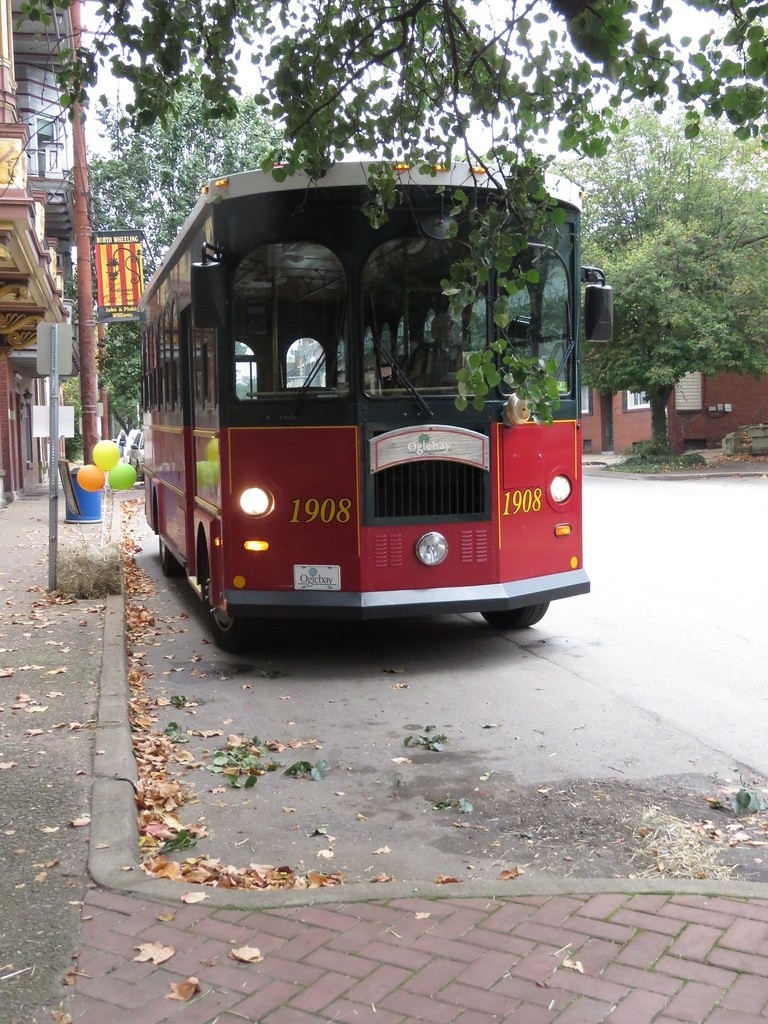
[196,437,219,488]
[93,439,120,471]
[77,464,106,492]
[108,462,138,491]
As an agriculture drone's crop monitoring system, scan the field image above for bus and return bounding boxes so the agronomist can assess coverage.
[136,162,615,651]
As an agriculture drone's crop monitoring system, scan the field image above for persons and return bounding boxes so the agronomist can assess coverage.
[400,313,474,396]
[119,429,126,441]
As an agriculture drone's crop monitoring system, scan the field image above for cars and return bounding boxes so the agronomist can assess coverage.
[113,429,145,479]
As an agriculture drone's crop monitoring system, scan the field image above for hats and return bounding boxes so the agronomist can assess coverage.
[431,313,455,328]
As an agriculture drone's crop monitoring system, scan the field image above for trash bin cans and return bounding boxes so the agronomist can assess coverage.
[65,467,104,524]
